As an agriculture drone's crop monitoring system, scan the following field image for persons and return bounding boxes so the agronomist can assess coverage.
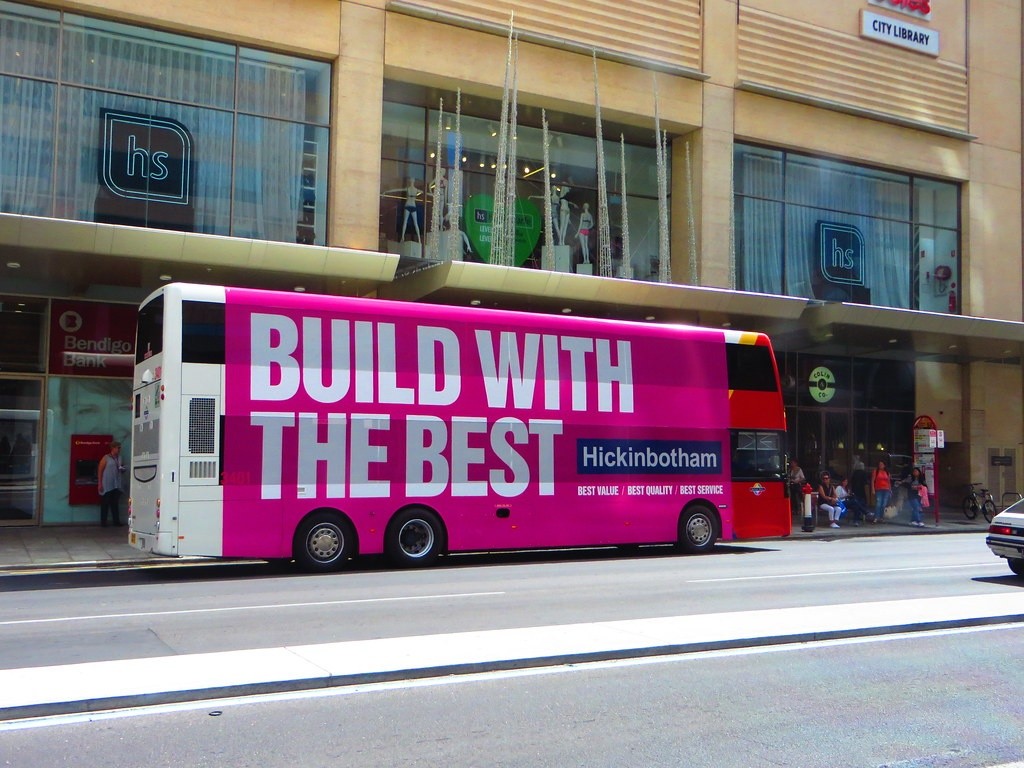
[528,184,594,264]
[383,168,473,254]
[787,460,928,528]
[97,441,126,527]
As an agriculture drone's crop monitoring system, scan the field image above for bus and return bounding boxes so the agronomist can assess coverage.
[128,282,792,573]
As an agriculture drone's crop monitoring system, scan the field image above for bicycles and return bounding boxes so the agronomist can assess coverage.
[962,482,997,523]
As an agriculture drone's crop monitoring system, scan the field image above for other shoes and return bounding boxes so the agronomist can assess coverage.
[911,521,924,527]
[854,522,859,527]
[869,513,875,519]
[829,522,840,529]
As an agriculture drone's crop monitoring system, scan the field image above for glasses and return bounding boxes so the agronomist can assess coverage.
[822,477,828,481]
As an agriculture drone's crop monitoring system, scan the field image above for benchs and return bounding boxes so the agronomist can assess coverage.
[811,491,865,527]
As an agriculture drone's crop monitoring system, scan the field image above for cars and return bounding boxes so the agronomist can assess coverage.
[985,499,1024,577]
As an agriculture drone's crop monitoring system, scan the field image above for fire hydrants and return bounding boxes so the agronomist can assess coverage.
[802,481,815,534]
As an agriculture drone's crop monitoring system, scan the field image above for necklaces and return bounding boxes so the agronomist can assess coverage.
[110,454,119,470]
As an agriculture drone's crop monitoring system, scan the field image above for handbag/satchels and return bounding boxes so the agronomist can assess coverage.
[883,492,898,519]
